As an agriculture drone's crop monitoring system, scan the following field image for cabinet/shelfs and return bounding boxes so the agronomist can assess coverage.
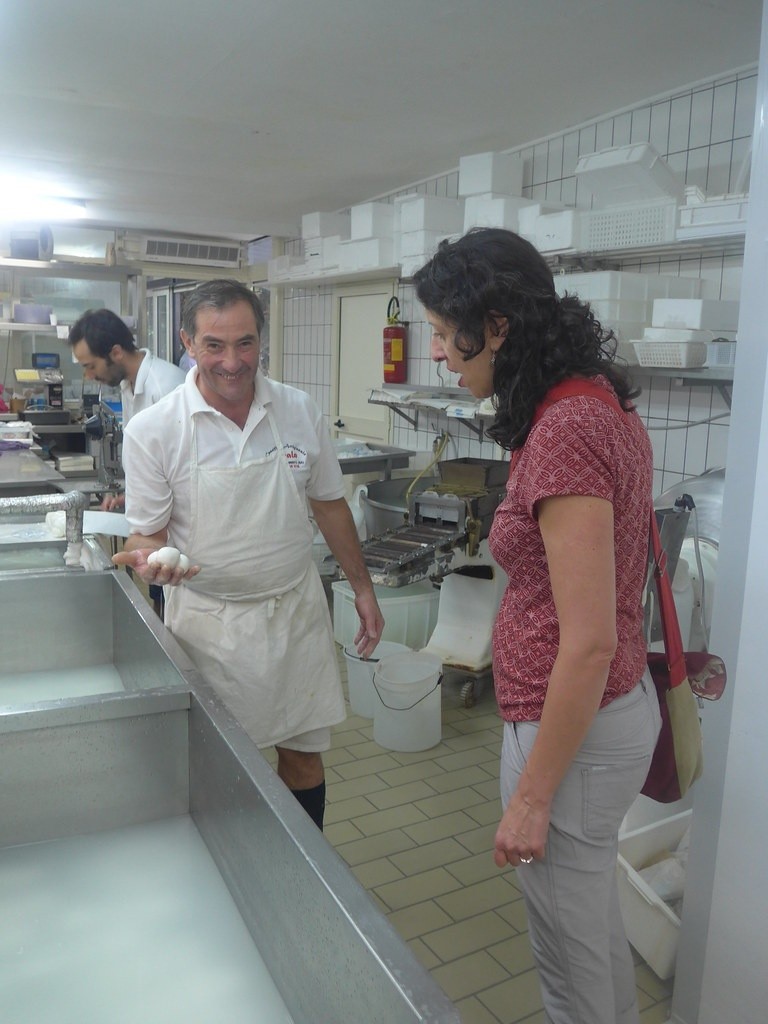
[0,258,148,478]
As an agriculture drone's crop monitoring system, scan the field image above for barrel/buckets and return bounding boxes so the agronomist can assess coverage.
[343,641,413,719]
[373,651,441,752]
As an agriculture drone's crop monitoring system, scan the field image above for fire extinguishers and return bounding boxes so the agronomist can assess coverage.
[382,295,409,385]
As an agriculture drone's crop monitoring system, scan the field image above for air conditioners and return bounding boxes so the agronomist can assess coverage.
[137,233,242,267]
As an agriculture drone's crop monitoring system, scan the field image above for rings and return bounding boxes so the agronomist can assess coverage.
[519,856,533,864]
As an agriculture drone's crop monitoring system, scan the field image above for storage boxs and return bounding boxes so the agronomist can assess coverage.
[643,329,737,343]
[268,152,577,284]
[331,579,440,650]
[551,269,702,367]
[652,299,738,331]
[617,808,693,981]
[578,199,679,253]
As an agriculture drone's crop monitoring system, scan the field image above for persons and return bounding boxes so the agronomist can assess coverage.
[66,306,186,623]
[178,349,198,374]
[122,278,385,834]
[408,224,671,1024]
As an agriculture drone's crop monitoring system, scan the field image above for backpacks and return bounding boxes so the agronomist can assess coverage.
[641,644,727,804]
